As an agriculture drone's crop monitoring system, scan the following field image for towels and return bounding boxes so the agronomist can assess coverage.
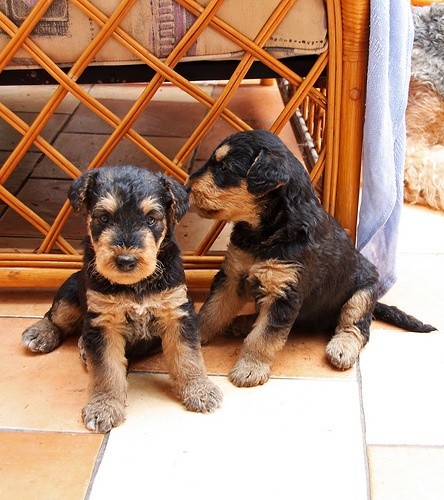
[353,0,414,299]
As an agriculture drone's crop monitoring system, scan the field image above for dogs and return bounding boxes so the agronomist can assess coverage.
[21,164,221,432]
[184,130,436,388]
